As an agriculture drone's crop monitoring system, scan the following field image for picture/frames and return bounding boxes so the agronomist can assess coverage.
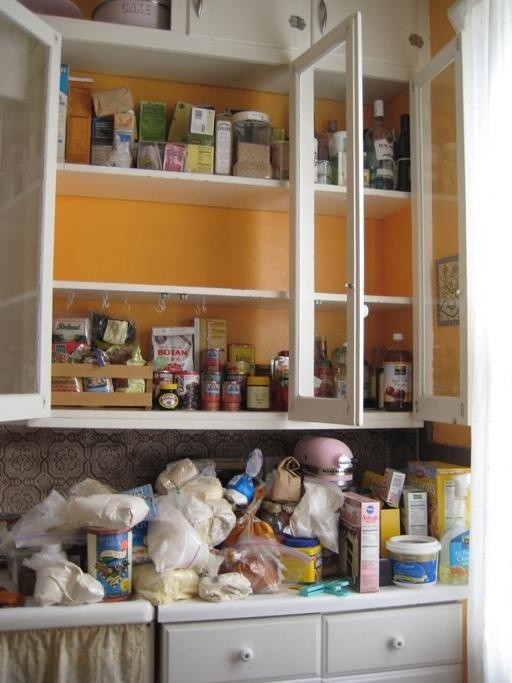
[435,254,460,325]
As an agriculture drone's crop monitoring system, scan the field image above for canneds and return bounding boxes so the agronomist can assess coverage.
[0,513,21,559]
[153,348,273,411]
[270,141,289,180]
[86,526,135,603]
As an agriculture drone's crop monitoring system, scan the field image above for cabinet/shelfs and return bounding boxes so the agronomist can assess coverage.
[0,0,466,430]
[324,603,466,683]
[171,0,312,50]
[312,0,430,64]
[163,614,324,683]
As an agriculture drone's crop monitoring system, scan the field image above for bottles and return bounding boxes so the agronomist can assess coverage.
[266,125,289,179]
[228,109,273,180]
[271,333,413,413]
[310,96,409,192]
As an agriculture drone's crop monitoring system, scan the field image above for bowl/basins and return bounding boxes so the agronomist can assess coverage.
[90,0,170,32]
